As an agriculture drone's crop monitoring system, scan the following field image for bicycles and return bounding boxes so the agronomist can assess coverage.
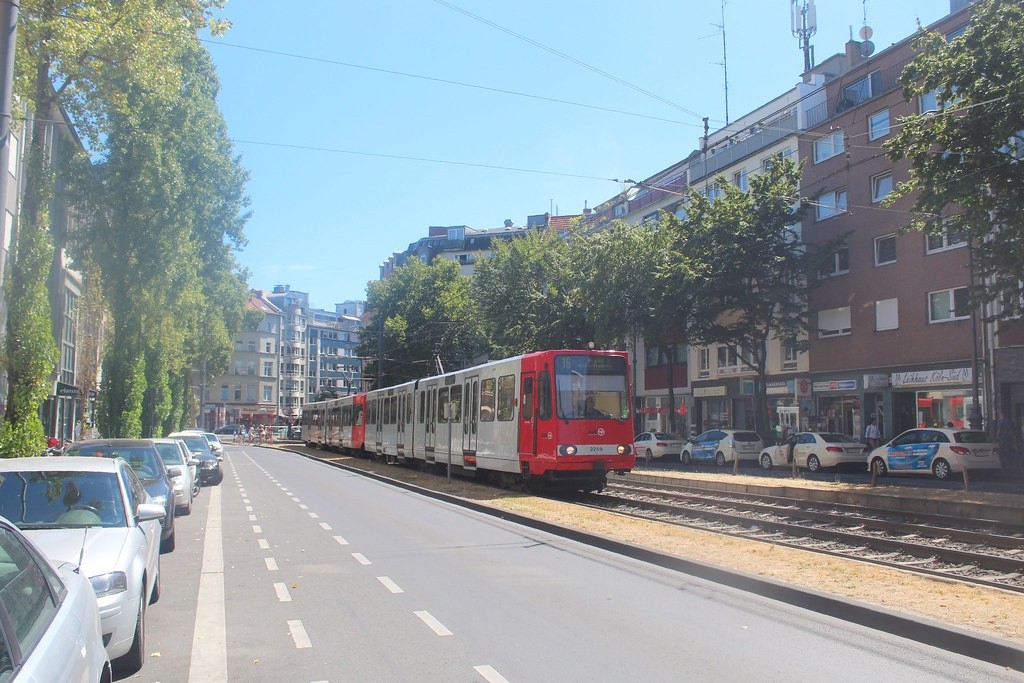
[243,434,261,446]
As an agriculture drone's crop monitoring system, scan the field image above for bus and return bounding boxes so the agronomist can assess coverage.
[301,342,635,494]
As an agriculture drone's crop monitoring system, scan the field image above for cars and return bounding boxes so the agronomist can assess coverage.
[0,456,166,674]
[633,429,688,463]
[139,438,200,515]
[63,437,182,553]
[866,428,1002,482]
[180,429,224,462]
[758,432,869,473]
[0,516,114,683]
[215,424,242,434]
[680,429,765,467]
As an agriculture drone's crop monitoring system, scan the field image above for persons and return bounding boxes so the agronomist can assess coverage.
[75,419,82,440]
[996,411,1010,452]
[581,397,604,417]
[865,417,883,450]
[238,424,266,446]
[946,422,954,428]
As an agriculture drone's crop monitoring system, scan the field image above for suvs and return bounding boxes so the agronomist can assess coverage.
[167,432,224,486]
